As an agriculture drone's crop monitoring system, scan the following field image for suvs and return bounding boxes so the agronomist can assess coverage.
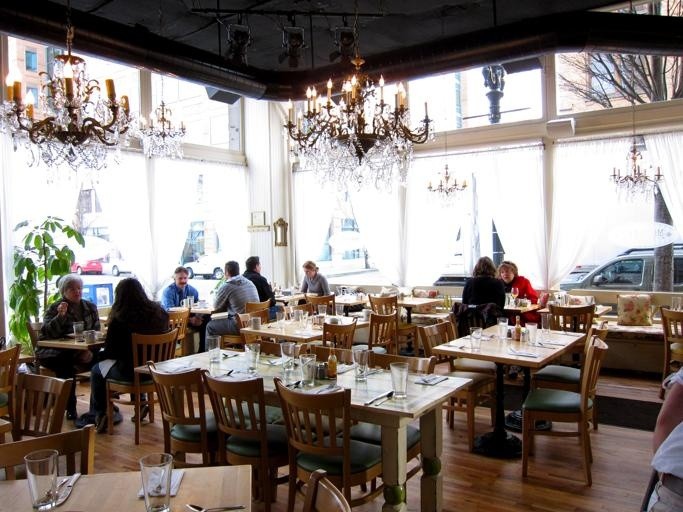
[556,242,682,295]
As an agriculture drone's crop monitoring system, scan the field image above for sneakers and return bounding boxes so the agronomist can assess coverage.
[131,401,148,421]
[95,415,107,433]
[67,409,77,420]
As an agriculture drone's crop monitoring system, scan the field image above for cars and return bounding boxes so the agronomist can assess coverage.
[432,272,476,288]
[111,249,183,279]
[565,265,596,284]
[182,251,228,280]
[67,259,103,276]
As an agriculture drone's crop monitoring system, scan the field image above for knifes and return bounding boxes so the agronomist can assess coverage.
[365,389,394,406]
[55,472,80,506]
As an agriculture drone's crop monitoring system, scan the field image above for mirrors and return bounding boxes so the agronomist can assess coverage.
[272,217,289,247]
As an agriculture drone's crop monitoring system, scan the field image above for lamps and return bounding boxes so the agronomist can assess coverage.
[138,0,189,164]
[426,148,468,197]
[604,1,665,200]
[275,0,437,195]
[0,0,138,175]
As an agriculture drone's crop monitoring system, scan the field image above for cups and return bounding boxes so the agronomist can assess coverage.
[182,296,206,310]
[670,296,680,311]
[251,317,261,330]
[84,330,96,344]
[275,304,371,334]
[23,449,58,512]
[206,334,407,402]
[72,322,84,342]
[470,313,553,352]
[139,452,173,512]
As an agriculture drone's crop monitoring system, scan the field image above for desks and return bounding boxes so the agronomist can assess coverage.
[3,464,253,509]
[431,321,586,462]
[37,331,106,352]
[134,348,474,511]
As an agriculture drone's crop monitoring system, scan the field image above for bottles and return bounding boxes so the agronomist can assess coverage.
[522,293,528,308]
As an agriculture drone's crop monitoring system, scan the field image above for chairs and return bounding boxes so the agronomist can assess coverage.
[274,345,353,436]
[202,369,319,510]
[167,282,682,358]
[337,352,437,496]
[1,425,97,482]
[658,304,683,400]
[273,379,384,510]
[416,320,498,445]
[302,469,351,511]
[148,361,252,469]
[1,343,20,424]
[229,340,308,424]
[531,321,600,433]
[520,335,609,486]
[2,372,75,481]
[26,322,41,350]
[105,326,180,446]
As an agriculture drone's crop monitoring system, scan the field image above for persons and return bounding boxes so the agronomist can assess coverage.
[35,273,104,420]
[298,260,329,305]
[90,278,169,433]
[242,256,278,320]
[457,256,505,338]
[645,365,683,512]
[161,265,205,357]
[495,261,542,329]
[205,261,260,352]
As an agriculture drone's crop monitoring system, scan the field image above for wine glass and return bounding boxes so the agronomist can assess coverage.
[511,288,519,308]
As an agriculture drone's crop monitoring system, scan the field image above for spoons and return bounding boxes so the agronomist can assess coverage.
[444,343,466,350]
[376,391,395,406]
[43,477,69,496]
[185,503,244,511]
[154,469,166,493]
